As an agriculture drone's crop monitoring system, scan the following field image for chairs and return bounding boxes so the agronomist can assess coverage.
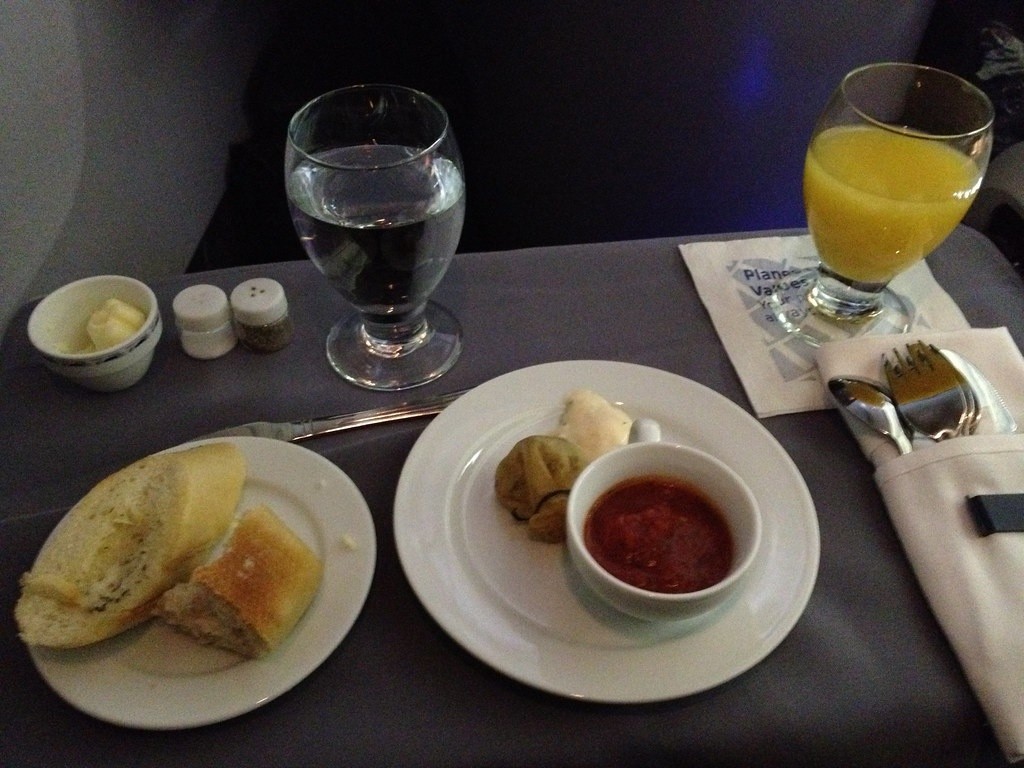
[441,0,1024,351]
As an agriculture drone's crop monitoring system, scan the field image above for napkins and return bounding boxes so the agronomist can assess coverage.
[813,327,1023,764]
[675,234,972,419]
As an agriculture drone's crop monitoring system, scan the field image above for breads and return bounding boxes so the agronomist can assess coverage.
[153,504,322,657]
[15,442,246,649]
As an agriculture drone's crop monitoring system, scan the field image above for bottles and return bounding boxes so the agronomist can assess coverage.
[230,279,293,354]
[174,285,238,359]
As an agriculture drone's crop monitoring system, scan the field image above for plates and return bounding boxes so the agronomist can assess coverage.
[17,438,376,731]
[392,357,824,705]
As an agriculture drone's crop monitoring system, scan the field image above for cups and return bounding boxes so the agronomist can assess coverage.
[565,417,764,621]
[27,274,163,391]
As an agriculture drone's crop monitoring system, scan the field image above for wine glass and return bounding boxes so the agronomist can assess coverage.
[282,82,464,391]
[768,59,993,366]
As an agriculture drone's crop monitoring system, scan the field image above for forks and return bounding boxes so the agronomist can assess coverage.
[883,341,983,444]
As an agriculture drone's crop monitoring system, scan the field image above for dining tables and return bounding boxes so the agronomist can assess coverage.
[0,223,1024,768]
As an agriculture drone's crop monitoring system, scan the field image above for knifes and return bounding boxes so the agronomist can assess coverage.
[938,349,1017,436]
[181,387,489,447]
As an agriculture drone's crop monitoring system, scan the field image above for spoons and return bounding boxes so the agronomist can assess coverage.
[828,374,914,456]
